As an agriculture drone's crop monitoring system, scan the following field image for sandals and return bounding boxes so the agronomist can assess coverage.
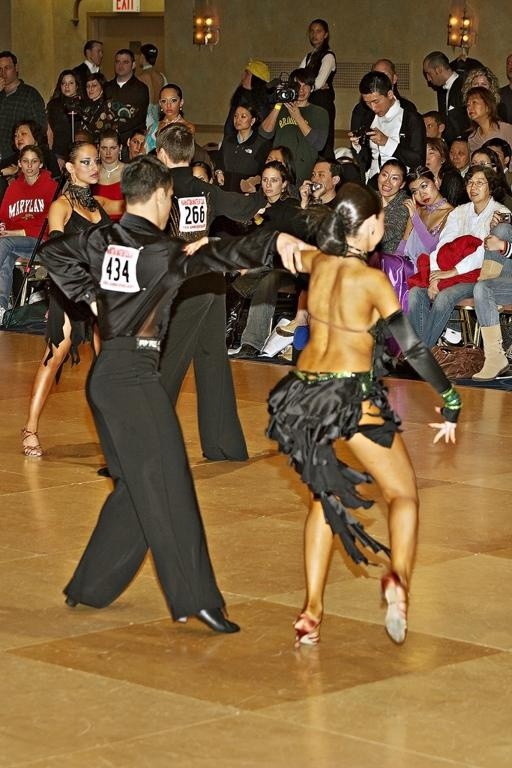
[24,431,43,457]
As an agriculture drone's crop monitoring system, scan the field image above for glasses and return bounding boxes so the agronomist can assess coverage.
[467,181,488,186]
[471,160,494,166]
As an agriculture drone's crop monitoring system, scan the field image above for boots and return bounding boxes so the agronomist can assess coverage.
[472,324,510,382]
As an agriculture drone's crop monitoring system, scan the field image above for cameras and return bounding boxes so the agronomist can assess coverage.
[352,126,374,144]
[61,96,82,113]
[310,183,321,192]
[497,213,511,223]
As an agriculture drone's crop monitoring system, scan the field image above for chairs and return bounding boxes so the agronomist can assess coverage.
[229,296,297,349]
[14,261,42,307]
[435,296,512,347]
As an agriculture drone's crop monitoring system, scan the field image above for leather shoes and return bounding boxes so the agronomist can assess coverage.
[177,607,240,633]
[234,344,258,359]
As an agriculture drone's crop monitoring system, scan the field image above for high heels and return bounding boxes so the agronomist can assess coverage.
[382,575,409,644]
[293,614,320,648]
[278,346,292,361]
[276,320,301,336]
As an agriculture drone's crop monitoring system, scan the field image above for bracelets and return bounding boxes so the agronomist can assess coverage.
[439,386,463,410]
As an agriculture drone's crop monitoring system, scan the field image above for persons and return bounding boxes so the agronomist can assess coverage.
[184,182,463,651]
[153,124,269,464]
[34,155,318,635]
[1,40,212,323]
[20,141,126,459]
[216,19,511,384]
